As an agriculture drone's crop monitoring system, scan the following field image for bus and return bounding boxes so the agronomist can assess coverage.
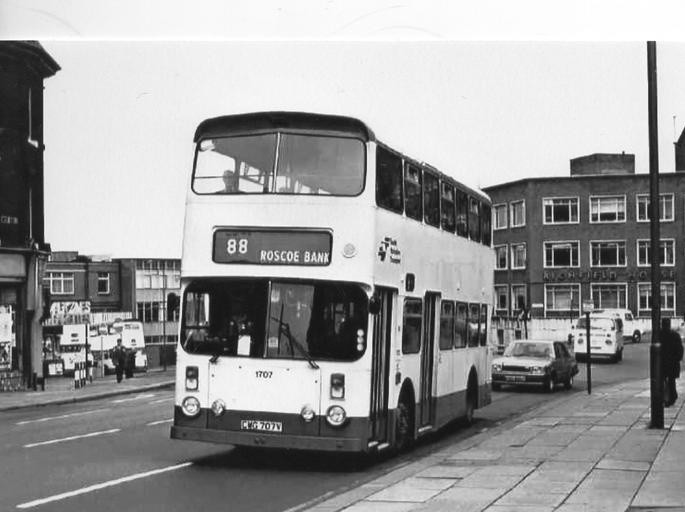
[169,111,495,459]
[43,298,94,378]
[90,318,147,376]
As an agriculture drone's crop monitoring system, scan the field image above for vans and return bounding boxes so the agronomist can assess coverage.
[570,308,647,344]
[571,313,624,364]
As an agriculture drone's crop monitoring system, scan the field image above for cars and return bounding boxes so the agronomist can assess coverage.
[490,339,580,393]
[672,320,685,343]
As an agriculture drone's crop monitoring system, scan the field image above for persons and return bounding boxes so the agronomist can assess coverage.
[111,339,127,382]
[659,318,683,406]
[213,170,245,193]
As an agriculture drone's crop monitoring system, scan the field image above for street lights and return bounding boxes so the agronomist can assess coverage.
[583,297,594,394]
